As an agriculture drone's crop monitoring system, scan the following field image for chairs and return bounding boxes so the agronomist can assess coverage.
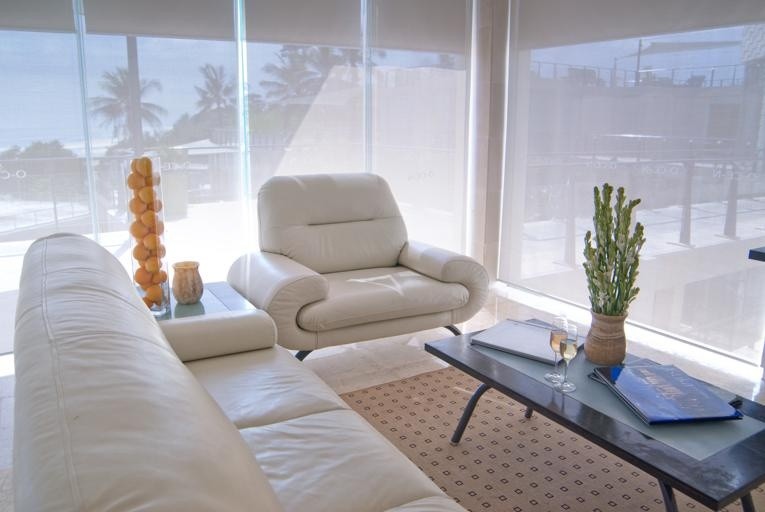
[227,174,489,361]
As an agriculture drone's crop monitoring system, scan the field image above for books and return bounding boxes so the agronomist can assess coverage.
[587,358,742,426]
[470,318,586,366]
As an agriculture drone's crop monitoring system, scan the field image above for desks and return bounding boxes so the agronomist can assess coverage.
[425,321,765,512]
[750,245,764,260]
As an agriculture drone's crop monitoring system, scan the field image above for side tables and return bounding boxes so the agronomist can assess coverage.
[138,281,254,321]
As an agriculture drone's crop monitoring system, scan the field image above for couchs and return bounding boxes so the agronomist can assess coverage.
[11,233,467,512]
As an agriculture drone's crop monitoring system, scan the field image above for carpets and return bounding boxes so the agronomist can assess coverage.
[338,365,765,512]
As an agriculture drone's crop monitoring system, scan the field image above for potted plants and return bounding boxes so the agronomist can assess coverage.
[582,183,647,364]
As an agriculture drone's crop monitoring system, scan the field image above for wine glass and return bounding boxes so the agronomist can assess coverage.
[544,317,568,383]
[554,325,578,393]
